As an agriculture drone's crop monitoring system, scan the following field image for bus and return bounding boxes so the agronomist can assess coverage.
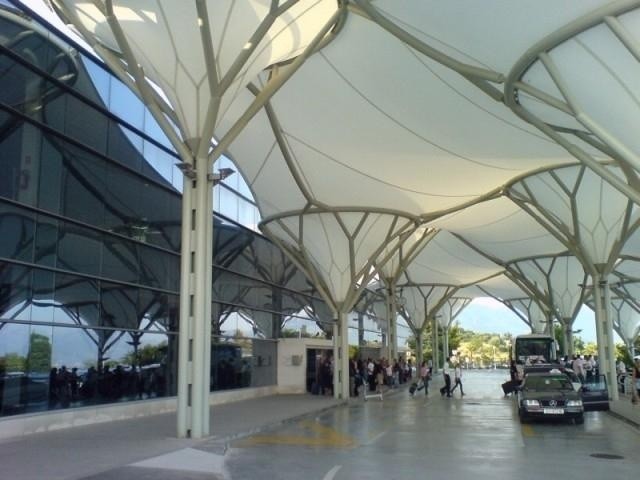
[512,334,560,380]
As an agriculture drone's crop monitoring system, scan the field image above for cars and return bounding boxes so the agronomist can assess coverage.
[513,365,610,427]
[0,371,49,407]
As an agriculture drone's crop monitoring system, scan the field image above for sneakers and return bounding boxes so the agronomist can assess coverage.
[439,387,466,398]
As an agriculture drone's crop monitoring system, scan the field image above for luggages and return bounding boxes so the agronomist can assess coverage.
[409,377,420,395]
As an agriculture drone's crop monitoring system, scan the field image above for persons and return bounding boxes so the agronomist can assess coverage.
[438,358,454,397]
[416,361,434,395]
[450,362,466,396]
[559,352,640,399]
[307,350,414,398]
[47,357,252,411]
[426,360,434,382]
[509,359,521,396]
